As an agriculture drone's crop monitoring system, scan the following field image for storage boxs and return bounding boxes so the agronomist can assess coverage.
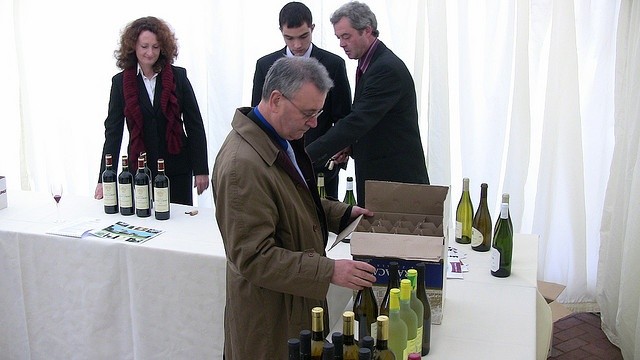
[329,180,452,325]
[536,280,574,358]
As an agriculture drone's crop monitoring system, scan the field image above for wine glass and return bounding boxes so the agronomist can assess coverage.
[47,180,66,223]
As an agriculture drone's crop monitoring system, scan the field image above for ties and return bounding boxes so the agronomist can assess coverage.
[287,141,309,187]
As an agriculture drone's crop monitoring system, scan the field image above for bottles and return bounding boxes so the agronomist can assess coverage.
[407,269,424,359]
[155,158,171,220]
[343,178,356,243]
[457,179,471,244]
[400,280,418,359]
[103,154,117,214]
[495,193,513,243]
[416,261,431,359]
[390,289,406,359]
[322,343,333,360]
[119,155,132,216]
[316,172,329,202]
[363,334,372,346]
[332,332,341,350]
[300,330,315,356]
[138,151,151,207]
[491,203,515,278]
[309,307,327,354]
[287,338,301,360]
[360,346,369,358]
[343,311,355,359]
[355,286,378,336]
[373,316,394,359]
[471,183,490,251]
[136,158,152,217]
[382,262,394,314]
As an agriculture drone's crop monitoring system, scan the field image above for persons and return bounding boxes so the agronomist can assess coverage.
[303,1,430,208]
[211,56,377,360]
[94,16,210,206]
[251,2,353,201]
